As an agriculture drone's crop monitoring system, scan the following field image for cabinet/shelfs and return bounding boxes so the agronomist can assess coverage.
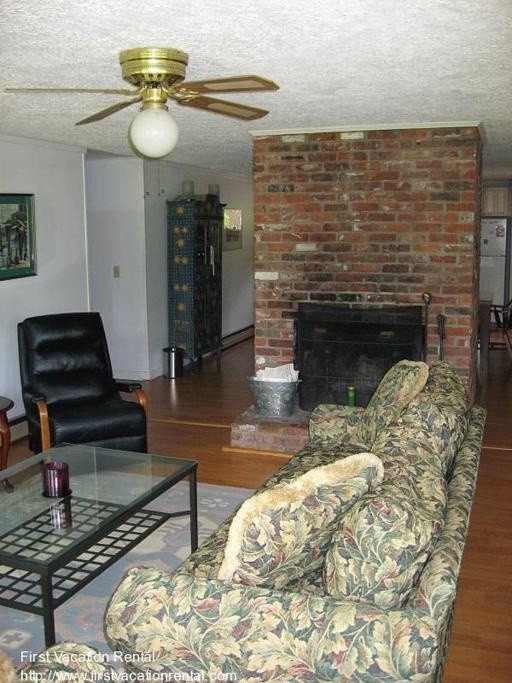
[167,200,223,358]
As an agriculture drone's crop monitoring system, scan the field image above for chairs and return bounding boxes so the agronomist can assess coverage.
[17,312,148,474]
[0,641,115,683]
[491,299,511,352]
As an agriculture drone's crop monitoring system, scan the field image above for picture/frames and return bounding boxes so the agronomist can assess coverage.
[0,193,37,280]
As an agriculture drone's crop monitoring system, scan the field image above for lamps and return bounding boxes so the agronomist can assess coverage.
[130,80,180,198]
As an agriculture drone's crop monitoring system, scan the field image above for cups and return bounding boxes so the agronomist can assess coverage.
[48,498,72,529]
[43,462,69,497]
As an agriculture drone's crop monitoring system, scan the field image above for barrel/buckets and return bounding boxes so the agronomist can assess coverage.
[247,377,301,419]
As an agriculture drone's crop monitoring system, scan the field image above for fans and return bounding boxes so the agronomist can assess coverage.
[3,48,280,126]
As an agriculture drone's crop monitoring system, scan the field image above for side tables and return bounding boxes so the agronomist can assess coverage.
[0,396,14,494]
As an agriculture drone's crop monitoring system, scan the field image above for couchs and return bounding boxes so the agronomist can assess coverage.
[106,360,488,682]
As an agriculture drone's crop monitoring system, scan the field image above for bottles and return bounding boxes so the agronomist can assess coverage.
[346,385,356,407]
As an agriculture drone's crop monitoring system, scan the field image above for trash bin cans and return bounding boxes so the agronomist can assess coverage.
[163,347,184,379]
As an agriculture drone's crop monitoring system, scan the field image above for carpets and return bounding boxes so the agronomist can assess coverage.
[1,471,258,673]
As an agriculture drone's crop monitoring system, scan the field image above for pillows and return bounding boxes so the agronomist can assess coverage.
[350,358,429,451]
[217,452,384,588]
